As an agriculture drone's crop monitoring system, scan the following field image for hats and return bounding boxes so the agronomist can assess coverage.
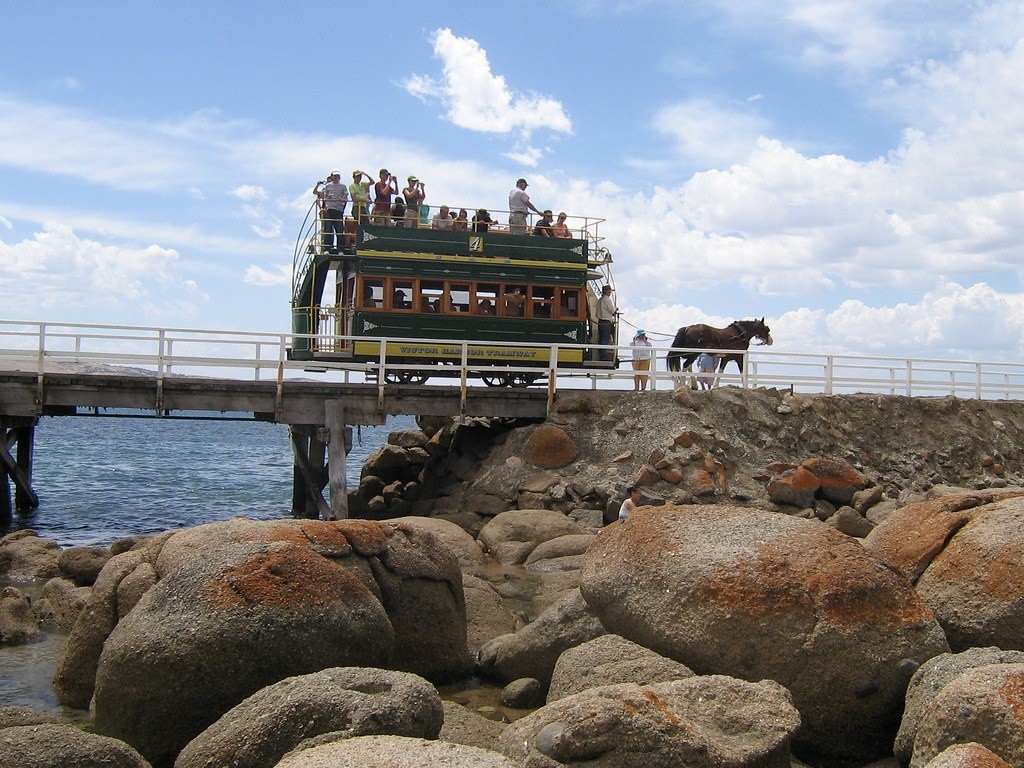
[397,291,407,297]
[637,330,646,336]
[600,285,615,292]
[331,169,340,176]
[408,176,418,181]
[353,170,361,177]
[517,178,529,186]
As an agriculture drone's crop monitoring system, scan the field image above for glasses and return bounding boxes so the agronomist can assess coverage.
[559,218,565,220]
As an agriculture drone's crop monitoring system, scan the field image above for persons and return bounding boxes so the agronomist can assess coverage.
[696,352,720,391]
[619,486,641,520]
[312,168,573,255]
[362,285,574,319]
[629,329,652,390]
[596,285,618,360]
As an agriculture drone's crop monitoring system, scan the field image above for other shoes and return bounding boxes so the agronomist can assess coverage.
[324,251,329,255]
[339,252,343,256]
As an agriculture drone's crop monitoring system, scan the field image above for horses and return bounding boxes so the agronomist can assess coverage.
[666,317,774,391]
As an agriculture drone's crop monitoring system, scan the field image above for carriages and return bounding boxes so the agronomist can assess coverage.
[285,197,773,389]
